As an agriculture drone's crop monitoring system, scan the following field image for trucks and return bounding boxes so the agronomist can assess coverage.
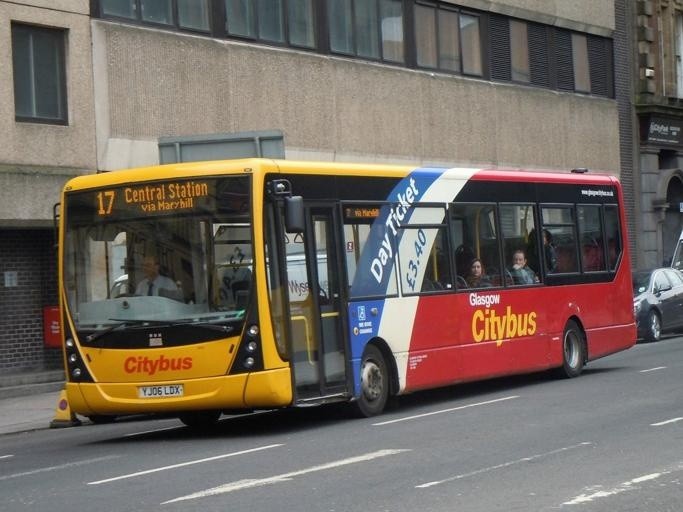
[668,227,682,274]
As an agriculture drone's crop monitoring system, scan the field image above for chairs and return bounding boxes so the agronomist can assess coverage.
[419,229,601,296]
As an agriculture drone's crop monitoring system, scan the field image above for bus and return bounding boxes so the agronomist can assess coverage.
[57,157,638,420]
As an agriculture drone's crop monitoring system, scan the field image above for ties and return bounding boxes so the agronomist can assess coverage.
[149,284,153,296]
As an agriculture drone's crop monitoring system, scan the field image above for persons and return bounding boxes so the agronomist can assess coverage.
[464,257,492,288]
[131,252,178,298]
[543,229,559,274]
[507,248,539,285]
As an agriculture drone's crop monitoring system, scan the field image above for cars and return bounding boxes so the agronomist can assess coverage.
[633,264,681,342]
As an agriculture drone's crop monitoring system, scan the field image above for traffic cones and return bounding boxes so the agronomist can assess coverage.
[49,387,81,429]
